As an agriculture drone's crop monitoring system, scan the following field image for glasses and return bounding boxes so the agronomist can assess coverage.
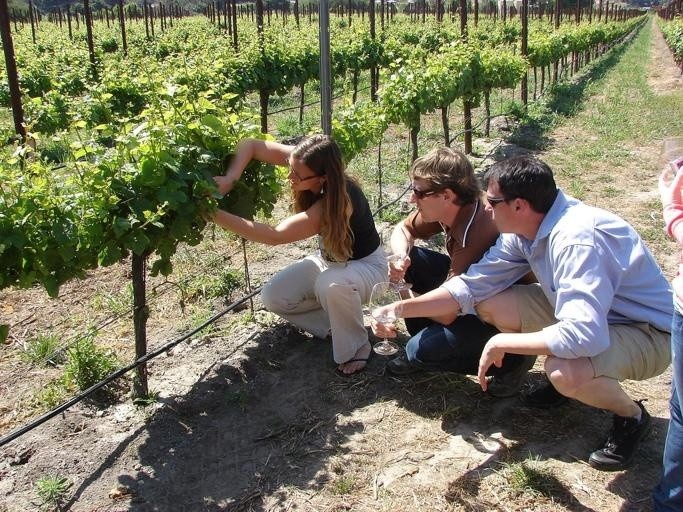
[292,168,318,182]
[487,197,505,206]
[413,189,435,198]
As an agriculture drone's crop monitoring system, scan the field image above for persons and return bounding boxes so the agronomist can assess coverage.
[370,156,673,472]
[198,134,388,378]
[650,160,683,512]
[385,146,539,398]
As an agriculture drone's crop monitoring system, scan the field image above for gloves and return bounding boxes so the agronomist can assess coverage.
[659,166,683,236]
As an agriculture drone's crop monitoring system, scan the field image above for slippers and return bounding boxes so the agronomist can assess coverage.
[335,359,368,377]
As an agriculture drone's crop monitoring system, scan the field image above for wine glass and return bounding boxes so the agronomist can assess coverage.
[664,137,683,207]
[368,280,403,357]
[382,238,413,292]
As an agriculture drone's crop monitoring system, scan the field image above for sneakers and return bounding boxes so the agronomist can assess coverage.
[589,399,651,471]
[488,352,568,407]
[385,356,437,384]
